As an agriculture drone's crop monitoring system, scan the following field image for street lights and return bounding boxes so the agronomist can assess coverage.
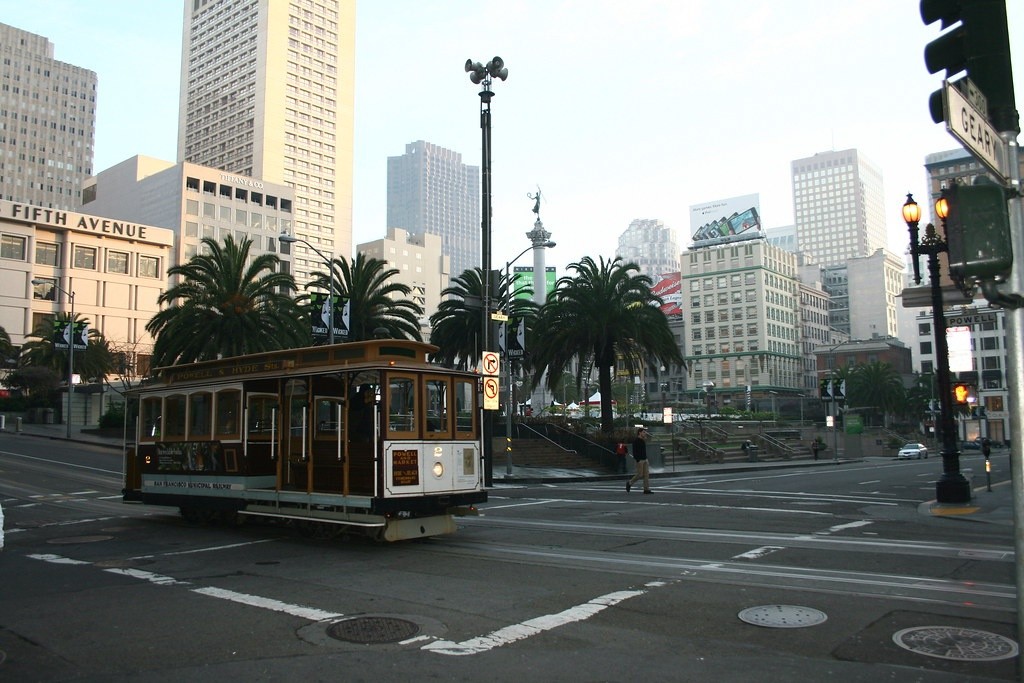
[278,235,335,345]
[902,183,973,506]
[828,339,864,461]
[492,241,557,354]
[464,55,508,486]
[31,279,75,439]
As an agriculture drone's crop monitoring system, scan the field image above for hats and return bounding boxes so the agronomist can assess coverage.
[747,440,751,443]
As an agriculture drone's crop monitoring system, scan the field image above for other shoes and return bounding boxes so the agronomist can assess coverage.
[626,482,630,492]
[644,491,654,494]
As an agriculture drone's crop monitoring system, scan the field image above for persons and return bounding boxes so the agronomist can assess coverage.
[812,439,819,460]
[741,440,751,456]
[614,440,628,474]
[626,428,654,494]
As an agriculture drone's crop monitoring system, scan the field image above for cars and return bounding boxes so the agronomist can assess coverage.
[975,436,994,447]
[964,441,981,451]
[897,443,929,460]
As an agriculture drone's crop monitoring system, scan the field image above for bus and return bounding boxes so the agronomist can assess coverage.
[120,338,489,543]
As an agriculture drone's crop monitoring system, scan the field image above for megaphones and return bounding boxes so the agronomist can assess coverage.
[464,56,508,84]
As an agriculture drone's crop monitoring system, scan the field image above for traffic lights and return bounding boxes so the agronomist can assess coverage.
[951,380,971,405]
[916,0,1014,125]
